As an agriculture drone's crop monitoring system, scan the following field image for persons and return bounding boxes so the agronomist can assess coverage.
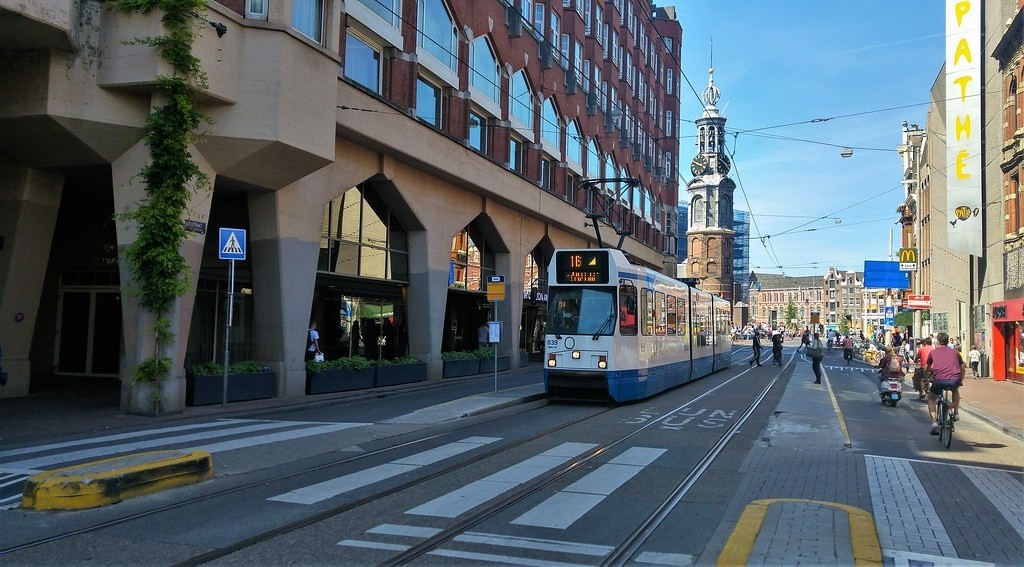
[878,331,910,371]
[827,329,841,349]
[916,335,962,363]
[749,332,763,367]
[807,333,824,384]
[797,332,810,352]
[361,317,397,353]
[876,343,906,382]
[305,321,321,362]
[349,320,361,354]
[914,337,935,398]
[477,321,489,348]
[843,335,853,361]
[731,320,772,341]
[860,331,864,340]
[537,327,542,349]
[923,333,965,433]
[969,345,981,379]
[772,334,783,368]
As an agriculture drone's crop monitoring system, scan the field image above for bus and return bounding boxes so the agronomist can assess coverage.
[530,176,732,406]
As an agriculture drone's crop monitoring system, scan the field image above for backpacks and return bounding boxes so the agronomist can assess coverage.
[887,358,901,372]
[306,331,315,350]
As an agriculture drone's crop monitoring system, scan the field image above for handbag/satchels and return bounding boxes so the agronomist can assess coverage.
[806,340,822,358]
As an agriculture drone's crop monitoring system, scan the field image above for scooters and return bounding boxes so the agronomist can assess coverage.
[873,364,903,408]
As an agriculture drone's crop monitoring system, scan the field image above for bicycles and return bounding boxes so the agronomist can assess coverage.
[842,346,853,365]
[826,337,833,350]
[914,358,934,398]
[922,377,963,449]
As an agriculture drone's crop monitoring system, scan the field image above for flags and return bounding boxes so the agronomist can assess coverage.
[749,272,757,283]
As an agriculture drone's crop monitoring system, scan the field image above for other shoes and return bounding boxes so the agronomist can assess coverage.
[749,361,752,366]
[814,380,822,384]
[931,422,939,431]
[954,414,959,421]
[757,364,762,366]
[977,373,979,377]
[873,389,881,395]
[974,377,975,379]
[773,364,776,367]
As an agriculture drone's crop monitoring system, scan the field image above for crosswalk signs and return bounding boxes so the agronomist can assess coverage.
[219,228,247,261]
